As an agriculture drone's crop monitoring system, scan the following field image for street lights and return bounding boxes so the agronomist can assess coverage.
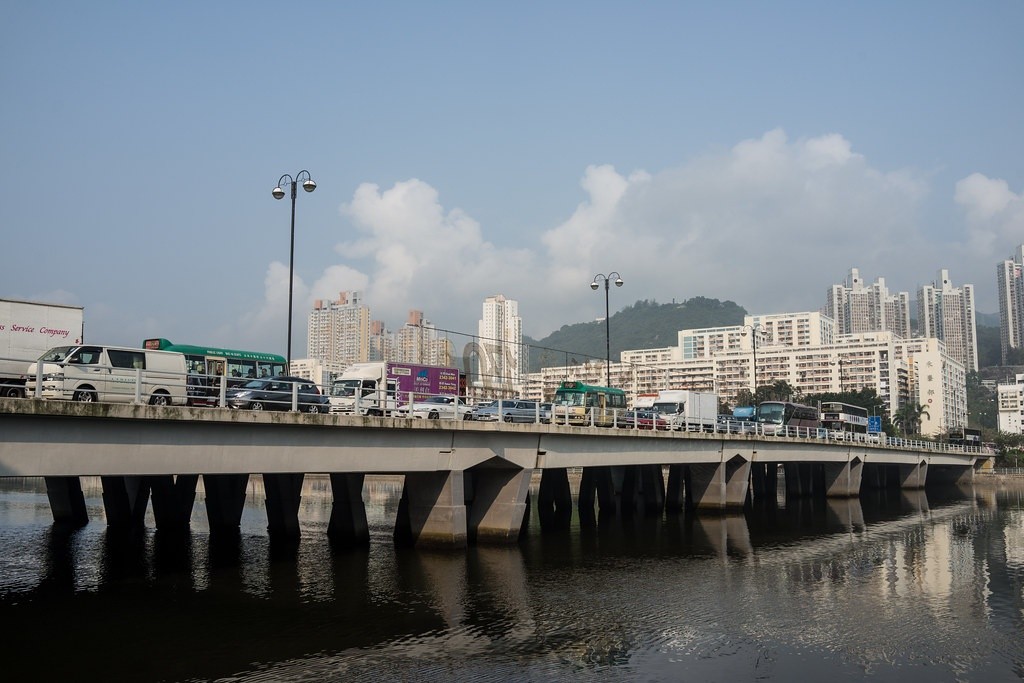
[830,356,852,403]
[272,170,316,376]
[741,324,767,421]
[591,272,624,388]
[874,405,881,432]
[979,413,986,447]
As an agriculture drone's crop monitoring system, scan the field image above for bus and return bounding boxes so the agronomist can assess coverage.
[142,338,288,407]
[717,401,868,442]
[553,381,627,426]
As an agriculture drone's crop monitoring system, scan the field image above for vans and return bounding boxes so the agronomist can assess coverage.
[25,345,187,406]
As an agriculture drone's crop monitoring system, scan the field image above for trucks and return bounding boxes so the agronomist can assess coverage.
[653,390,718,433]
[0,298,84,397]
[329,361,460,417]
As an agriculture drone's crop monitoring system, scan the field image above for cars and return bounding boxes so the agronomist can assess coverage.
[618,412,667,431]
[474,400,552,424]
[218,376,331,414]
[395,395,478,421]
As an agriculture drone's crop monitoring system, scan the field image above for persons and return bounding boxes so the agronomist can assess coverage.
[279,372,283,376]
[232,369,237,377]
[246,369,256,378]
[366,384,374,393]
[262,369,268,377]
[215,364,222,384]
[237,372,241,377]
